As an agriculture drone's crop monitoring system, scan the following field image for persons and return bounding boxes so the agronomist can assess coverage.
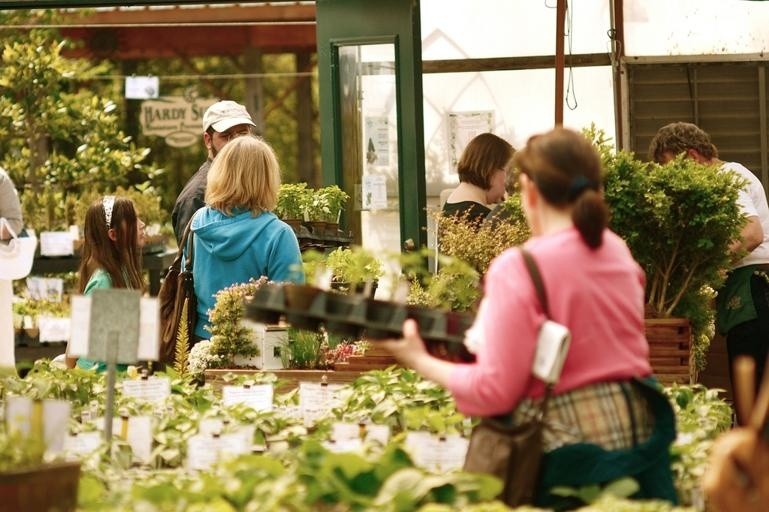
[0,166,26,367]
[170,98,260,243]
[178,135,305,348]
[647,119,769,425]
[382,120,684,512]
[64,192,147,371]
[434,132,521,273]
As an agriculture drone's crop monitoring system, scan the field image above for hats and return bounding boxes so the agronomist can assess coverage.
[202,100,257,133]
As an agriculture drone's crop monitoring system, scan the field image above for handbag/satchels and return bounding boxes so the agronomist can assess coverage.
[0,217,37,279]
[464,423,544,508]
[158,266,197,369]
